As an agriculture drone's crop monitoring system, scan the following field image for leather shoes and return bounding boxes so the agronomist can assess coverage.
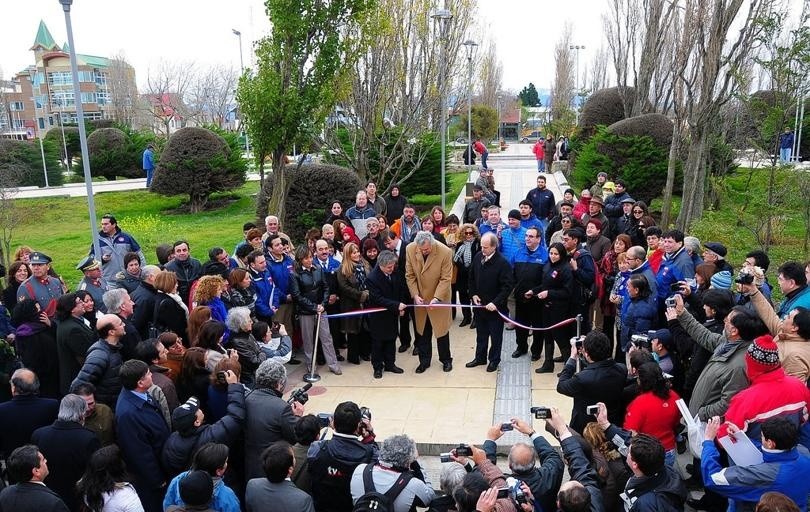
[682,464,704,510]
[316,343,452,379]
[460,315,565,373]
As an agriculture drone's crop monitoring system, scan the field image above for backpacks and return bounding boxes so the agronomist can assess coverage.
[354,462,416,512]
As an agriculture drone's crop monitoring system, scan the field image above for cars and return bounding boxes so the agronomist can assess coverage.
[457,136,469,143]
[520,130,543,144]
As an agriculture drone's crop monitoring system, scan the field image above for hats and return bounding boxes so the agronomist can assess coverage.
[745,334,780,378]
[704,243,727,257]
[648,329,672,345]
[738,265,765,288]
[508,210,521,220]
[171,396,200,431]
[621,197,636,203]
[561,203,574,208]
[29,252,52,265]
[564,189,574,196]
[160,333,177,347]
[587,196,606,208]
[178,471,213,505]
[76,257,101,271]
[710,271,732,289]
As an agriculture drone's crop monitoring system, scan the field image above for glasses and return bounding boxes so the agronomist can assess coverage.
[634,209,648,214]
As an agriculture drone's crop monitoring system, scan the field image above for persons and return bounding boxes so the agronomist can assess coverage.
[2,242,435,512]
[532,137,546,172]
[143,144,157,188]
[465,231,514,372]
[89,214,146,290]
[428,171,809,512]
[462,145,476,165]
[404,230,455,373]
[472,141,488,169]
[243,182,484,330]
[476,170,497,206]
[542,134,557,173]
[366,249,406,379]
[526,176,556,229]
[463,186,491,224]
[384,184,408,228]
[779,127,794,165]
[486,167,501,208]
[556,135,572,160]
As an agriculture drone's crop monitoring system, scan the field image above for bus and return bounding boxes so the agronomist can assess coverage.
[518,118,577,143]
[0,131,34,142]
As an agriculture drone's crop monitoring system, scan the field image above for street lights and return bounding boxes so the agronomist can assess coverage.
[462,33,478,187]
[58,0,101,275]
[432,7,452,214]
[339,113,343,128]
[569,41,585,129]
[231,26,250,159]
[55,100,72,177]
[497,94,505,142]
[23,64,52,188]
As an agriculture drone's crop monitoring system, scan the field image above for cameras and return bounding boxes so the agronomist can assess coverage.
[531,406,552,419]
[317,412,334,428]
[666,298,677,307]
[586,405,600,415]
[502,422,513,431]
[217,371,230,381]
[359,407,372,428]
[456,443,473,456]
[735,273,754,285]
[227,349,235,359]
[270,321,281,334]
[515,489,528,507]
[497,488,509,499]
[440,452,455,463]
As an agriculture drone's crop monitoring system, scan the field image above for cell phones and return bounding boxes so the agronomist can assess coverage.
[576,341,582,355]
[670,283,682,292]
[720,415,725,424]
